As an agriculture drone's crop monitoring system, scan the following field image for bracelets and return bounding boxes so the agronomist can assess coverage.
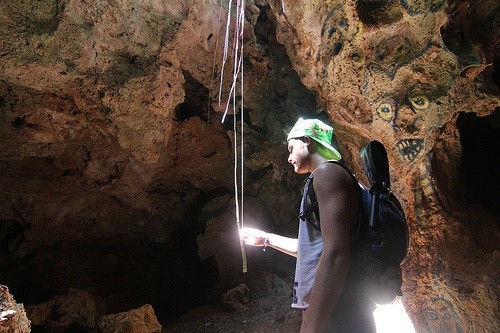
[263,230,268,251]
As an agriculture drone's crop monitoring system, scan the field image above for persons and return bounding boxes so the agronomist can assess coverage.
[238,116,376,332]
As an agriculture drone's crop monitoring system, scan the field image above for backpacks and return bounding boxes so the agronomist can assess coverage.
[300,142,411,303]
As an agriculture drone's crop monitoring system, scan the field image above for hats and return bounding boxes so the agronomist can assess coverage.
[287,119,342,163]
